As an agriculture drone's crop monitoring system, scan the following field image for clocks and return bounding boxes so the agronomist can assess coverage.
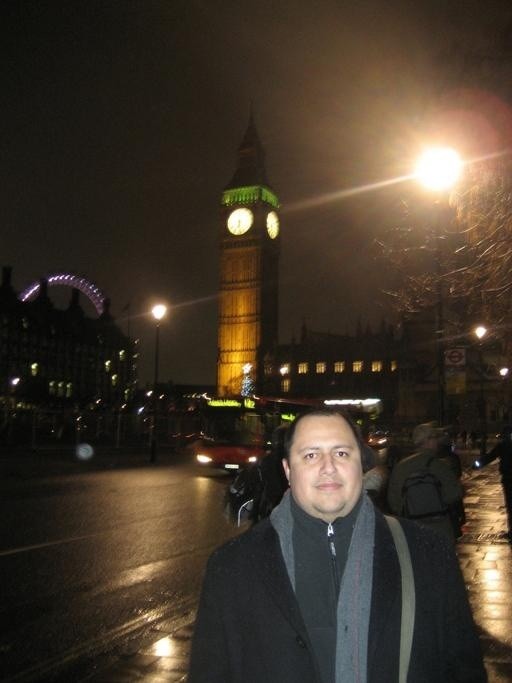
[226,207,254,235]
[266,211,280,240]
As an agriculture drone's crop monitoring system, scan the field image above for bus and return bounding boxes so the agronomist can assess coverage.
[196,393,363,475]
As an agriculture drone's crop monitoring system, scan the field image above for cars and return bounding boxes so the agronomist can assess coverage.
[367,429,393,447]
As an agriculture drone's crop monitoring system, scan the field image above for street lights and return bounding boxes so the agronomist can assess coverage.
[475,326,487,455]
[418,148,459,442]
[150,304,168,460]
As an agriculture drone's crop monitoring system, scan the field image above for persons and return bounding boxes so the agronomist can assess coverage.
[387,421,462,546]
[435,436,468,542]
[362,444,400,513]
[249,421,289,526]
[185,404,487,683]
[473,419,512,543]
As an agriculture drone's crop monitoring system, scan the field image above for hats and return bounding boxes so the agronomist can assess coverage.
[410,422,446,445]
